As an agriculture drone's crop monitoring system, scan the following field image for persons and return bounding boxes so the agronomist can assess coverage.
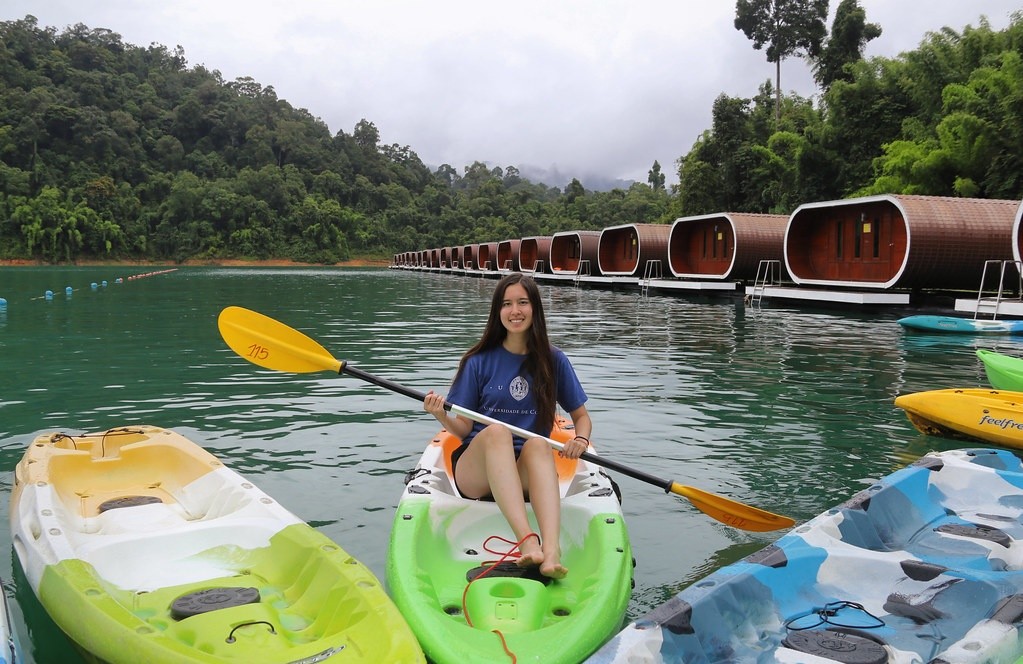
[424,272,592,579]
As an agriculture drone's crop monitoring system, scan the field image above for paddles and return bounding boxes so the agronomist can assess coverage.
[217,304,798,533]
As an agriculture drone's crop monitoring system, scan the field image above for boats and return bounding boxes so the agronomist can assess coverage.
[389,413,637,664]
[977,349,1023,392]
[893,389,1023,450]
[8,424,426,664]
[896,315,1023,336]
[580,448,1023,664]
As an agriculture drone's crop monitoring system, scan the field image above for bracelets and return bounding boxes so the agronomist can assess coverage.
[574,436,589,446]
